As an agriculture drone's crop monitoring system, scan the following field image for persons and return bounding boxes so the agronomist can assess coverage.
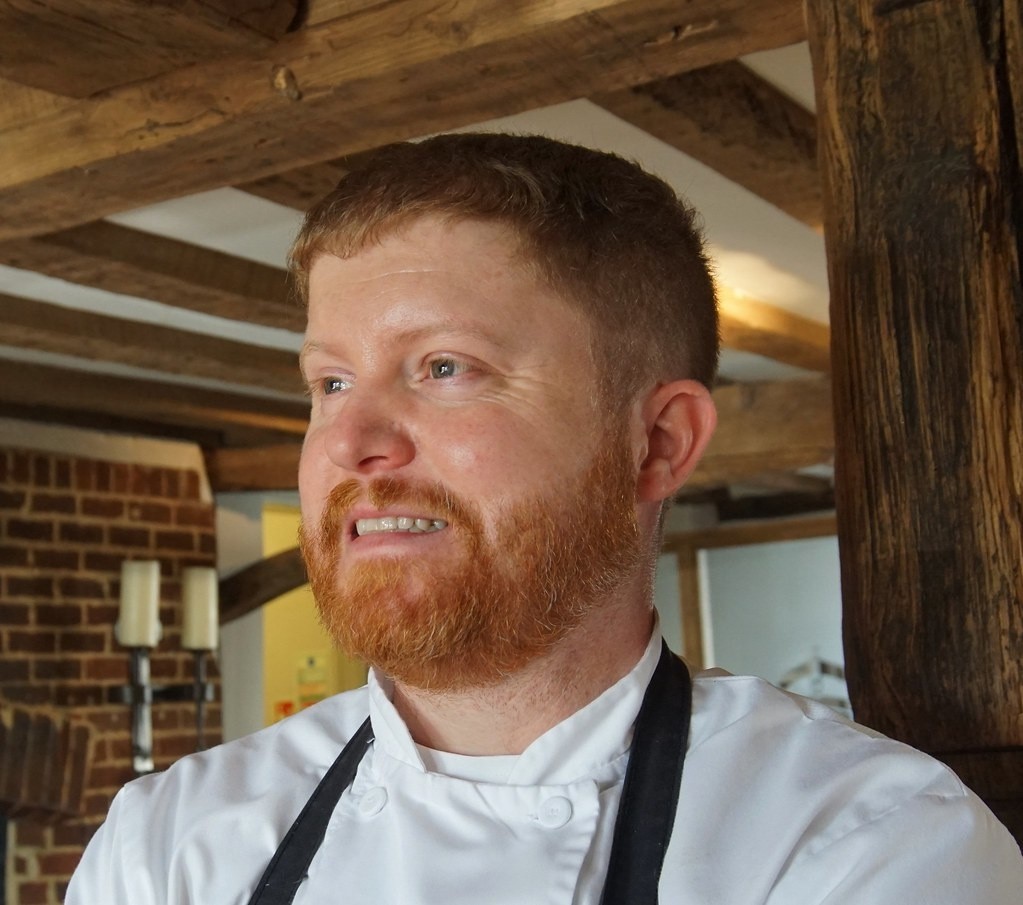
[64,133,1023,905]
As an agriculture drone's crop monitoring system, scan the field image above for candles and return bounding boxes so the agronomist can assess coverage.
[180,566,218,650]
[118,561,160,648]
